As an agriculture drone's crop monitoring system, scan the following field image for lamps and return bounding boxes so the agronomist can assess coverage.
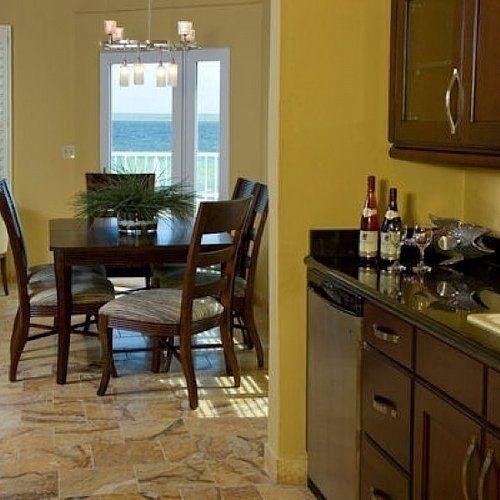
[97,0,206,88]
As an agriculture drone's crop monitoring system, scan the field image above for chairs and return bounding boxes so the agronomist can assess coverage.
[153,177,267,373]
[97,196,252,410]
[1,172,118,383]
[86,173,156,219]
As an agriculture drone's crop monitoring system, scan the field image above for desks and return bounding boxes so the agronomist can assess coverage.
[49,218,240,386]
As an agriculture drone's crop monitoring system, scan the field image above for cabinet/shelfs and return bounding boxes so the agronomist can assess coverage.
[355,300,498,499]
[386,1,498,170]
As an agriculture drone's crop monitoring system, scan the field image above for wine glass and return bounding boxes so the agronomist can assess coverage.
[412,273,431,311]
[411,226,434,272]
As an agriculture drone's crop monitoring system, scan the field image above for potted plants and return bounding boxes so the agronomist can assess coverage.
[69,173,202,237]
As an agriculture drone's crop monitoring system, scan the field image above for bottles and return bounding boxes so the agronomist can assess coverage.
[357,174,379,266]
[357,267,401,305]
[380,186,403,268]
